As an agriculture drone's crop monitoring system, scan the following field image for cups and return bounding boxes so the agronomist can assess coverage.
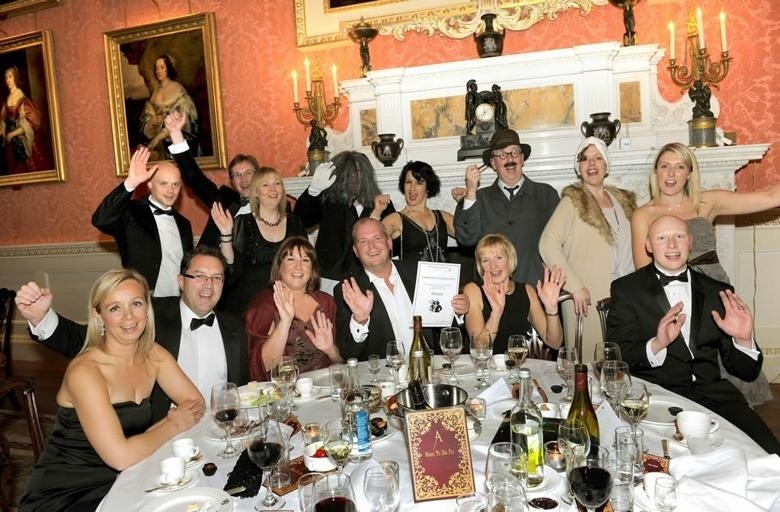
[157,436,200,482]
[677,410,719,441]
[644,471,676,498]
[657,477,680,512]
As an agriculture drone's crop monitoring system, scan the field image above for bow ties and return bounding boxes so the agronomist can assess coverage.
[153,207,172,217]
[191,315,215,329]
[659,271,689,286]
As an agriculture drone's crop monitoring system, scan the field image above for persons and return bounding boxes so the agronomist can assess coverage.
[15,110,568,511]
[632,142,780,410]
[491,84,508,130]
[601,214,779,458]
[465,83,480,135]
[139,53,200,160]
[1,66,55,175]
[538,135,638,359]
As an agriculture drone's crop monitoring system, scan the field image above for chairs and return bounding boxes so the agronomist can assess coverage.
[557,291,583,363]
[595,296,613,343]
[0,375,47,465]
[0,287,24,412]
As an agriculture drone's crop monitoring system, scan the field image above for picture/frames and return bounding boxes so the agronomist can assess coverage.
[293,1,614,48]
[0,29,67,187]
[101,10,229,177]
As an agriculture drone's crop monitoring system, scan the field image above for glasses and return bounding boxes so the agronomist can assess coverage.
[498,150,519,160]
[182,271,224,286]
[231,168,256,182]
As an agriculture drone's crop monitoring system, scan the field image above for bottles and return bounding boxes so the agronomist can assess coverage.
[408,316,432,386]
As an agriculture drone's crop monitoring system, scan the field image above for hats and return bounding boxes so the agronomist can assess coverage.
[482,129,531,168]
[575,135,611,175]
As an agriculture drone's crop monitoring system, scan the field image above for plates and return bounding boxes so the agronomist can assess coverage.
[636,399,690,427]
[201,415,264,441]
[151,452,206,491]
[131,486,235,512]
[665,428,725,448]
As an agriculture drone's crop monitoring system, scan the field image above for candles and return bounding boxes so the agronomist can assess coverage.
[695,7,706,49]
[302,56,312,93]
[719,8,728,53]
[667,20,676,58]
[290,70,300,103]
[331,63,338,96]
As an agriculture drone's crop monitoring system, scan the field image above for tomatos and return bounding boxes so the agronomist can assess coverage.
[313,448,326,457]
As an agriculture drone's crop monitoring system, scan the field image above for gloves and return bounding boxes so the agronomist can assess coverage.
[308,161,338,198]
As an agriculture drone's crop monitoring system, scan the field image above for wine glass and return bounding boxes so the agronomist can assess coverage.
[210,328,649,512]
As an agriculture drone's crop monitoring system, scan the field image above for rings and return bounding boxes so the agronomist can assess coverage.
[739,308,743,311]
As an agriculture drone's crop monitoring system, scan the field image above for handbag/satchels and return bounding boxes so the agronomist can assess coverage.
[223,441,262,498]
[492,410,566,450]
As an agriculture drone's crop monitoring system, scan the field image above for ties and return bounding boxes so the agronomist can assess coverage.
[504,186,519,200]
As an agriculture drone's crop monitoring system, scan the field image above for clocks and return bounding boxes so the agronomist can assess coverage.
[455,79,509,161]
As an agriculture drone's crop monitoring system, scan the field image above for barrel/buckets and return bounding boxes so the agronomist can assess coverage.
[387,385,469,447]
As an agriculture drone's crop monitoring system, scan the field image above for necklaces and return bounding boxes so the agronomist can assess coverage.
[584,189,620,234]
[659,192,685,211]
